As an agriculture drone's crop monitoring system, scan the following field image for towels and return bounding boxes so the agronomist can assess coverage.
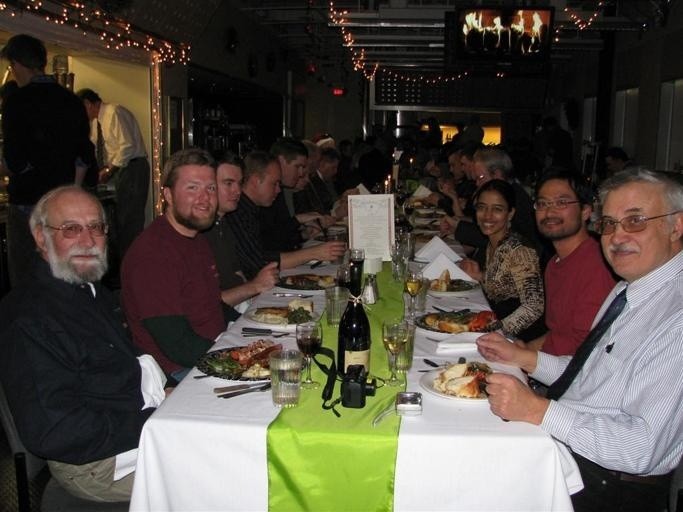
[412,235,463,264]
[433,328,514,357]
[413,251,478,287]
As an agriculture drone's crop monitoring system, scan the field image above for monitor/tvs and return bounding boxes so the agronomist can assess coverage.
[444,11,552,80]
[455,5,555,63]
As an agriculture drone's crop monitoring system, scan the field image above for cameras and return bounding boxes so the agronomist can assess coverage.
[340,364,377,409]
[395,392,423,416]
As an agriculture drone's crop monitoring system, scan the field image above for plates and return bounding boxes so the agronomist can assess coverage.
[416,309,506,343]
[417,362,505,405]
[198,345,314,383]
[274,273,339,290]
[427,278,481,298]
[301,197,472,266]
[243,306,321,324]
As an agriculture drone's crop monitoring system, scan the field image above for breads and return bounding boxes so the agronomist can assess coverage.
[438,269,451,292]
[289,299,314,313]
[432,363,480,398]
[425,314,469,333]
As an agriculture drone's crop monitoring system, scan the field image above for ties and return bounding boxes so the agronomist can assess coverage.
[548,287,627,399]
[96,120,107,168]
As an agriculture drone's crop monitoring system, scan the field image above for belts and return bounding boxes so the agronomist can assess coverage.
[586,469,667,487]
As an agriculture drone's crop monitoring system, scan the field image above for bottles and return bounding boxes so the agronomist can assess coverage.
[337,247,371,380]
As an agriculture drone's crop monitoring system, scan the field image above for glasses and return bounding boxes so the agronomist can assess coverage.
[474,205,511,214]
[43,222,108,237]
[593,210,683,234]
[532,199,582,209]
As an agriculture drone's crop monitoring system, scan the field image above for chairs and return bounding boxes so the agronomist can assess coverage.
[0,379,133,511]
[586,455,682,512]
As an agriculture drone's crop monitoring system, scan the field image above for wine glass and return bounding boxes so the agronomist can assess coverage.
[381,318,410,390]
[296,322,323,391]
[402,271,424,317]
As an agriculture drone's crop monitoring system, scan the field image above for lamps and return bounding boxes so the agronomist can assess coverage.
[454,0,556,82]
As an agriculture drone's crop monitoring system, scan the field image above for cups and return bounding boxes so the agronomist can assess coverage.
[269,347,301,407]
[325,285,350,328]
